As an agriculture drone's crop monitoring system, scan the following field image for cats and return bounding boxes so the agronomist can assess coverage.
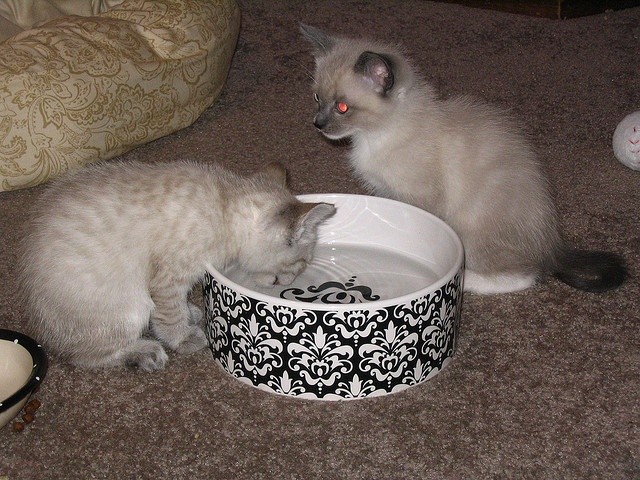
[299,23,637,295]
[17,155,338,372]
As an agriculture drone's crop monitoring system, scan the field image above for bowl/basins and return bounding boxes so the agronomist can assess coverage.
[200,192,466,403]
[0,327,50,430]
